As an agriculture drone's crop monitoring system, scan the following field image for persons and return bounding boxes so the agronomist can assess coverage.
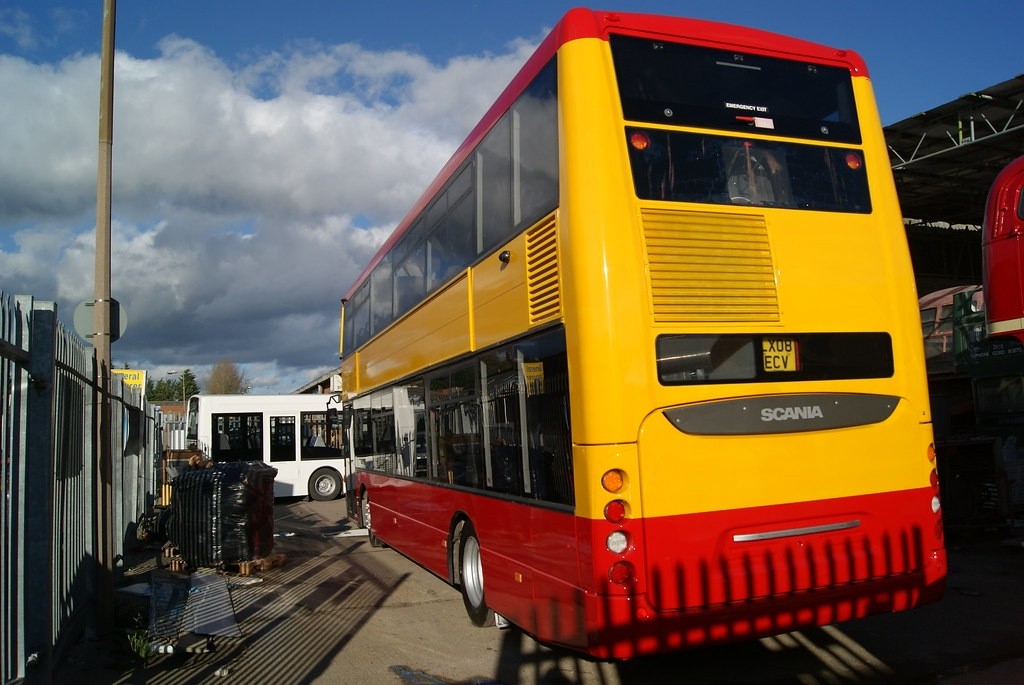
[183,455,215,472]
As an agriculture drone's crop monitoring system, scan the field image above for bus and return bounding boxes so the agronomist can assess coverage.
[326,7,947,662]
[185,385,495,501]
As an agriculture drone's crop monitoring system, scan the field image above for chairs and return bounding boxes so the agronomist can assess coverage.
[448,443,553,502]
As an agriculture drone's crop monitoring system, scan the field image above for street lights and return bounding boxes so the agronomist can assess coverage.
[167,371,185,415]
